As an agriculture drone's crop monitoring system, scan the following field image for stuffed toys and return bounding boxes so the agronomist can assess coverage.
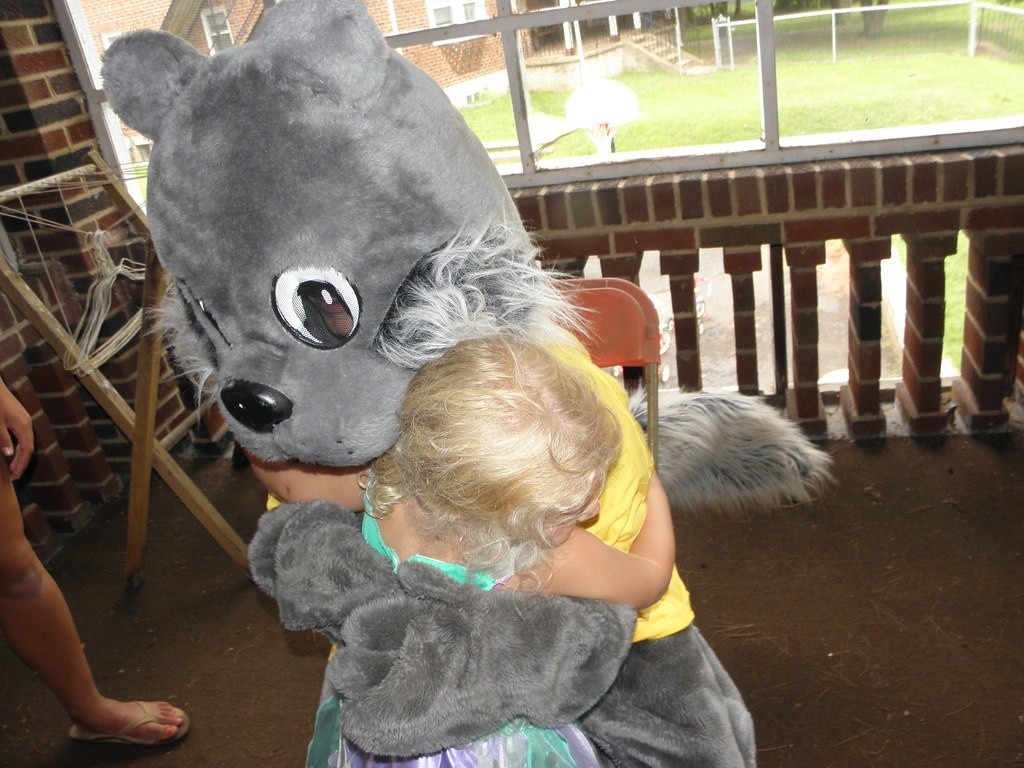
[103,0,758,768]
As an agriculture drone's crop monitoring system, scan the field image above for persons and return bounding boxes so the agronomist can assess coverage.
[246,333,676,768]
[1,378,192,749]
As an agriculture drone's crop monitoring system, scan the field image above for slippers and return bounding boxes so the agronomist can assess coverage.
[68,700,191,747]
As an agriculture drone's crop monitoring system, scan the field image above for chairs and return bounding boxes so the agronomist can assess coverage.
[552,279,662,475]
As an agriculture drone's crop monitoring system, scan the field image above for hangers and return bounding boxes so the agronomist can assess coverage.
[63,231,146,377]
